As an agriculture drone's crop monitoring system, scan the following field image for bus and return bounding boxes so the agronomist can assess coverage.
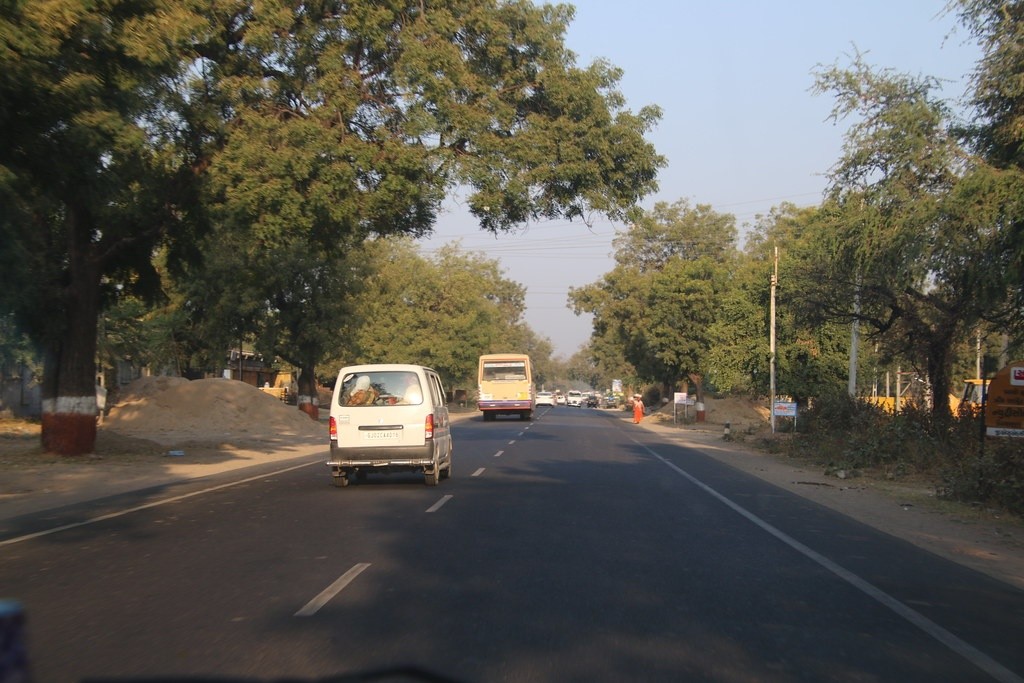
[475,353,539,421]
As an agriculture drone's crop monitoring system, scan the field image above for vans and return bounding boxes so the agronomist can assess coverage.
[324,364,455,488]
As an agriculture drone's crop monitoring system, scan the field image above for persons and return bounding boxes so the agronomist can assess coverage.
[348,374,375,406]
[633,397,645,424]
[396,374,422,405]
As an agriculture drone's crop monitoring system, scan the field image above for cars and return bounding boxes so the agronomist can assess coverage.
[536,389,619,409]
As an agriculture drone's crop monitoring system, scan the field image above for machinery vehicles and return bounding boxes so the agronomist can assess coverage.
[900,375,995,419]
